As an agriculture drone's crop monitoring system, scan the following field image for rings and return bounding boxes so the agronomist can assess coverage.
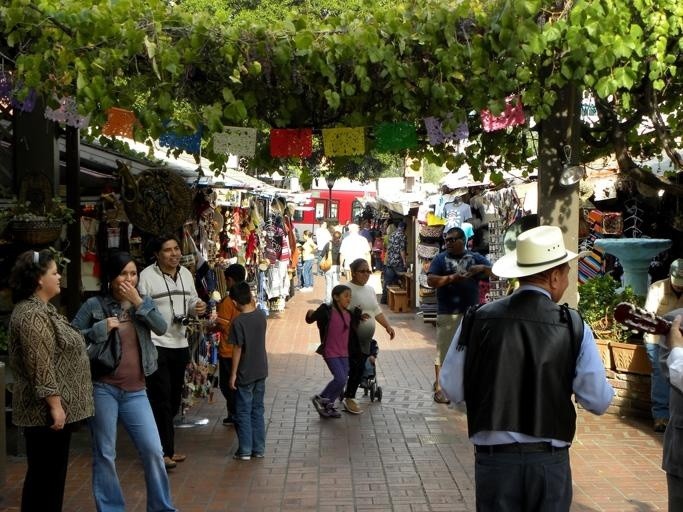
[122,289,126,292]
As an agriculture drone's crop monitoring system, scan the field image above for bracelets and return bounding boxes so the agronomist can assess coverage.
[447,274,452,282]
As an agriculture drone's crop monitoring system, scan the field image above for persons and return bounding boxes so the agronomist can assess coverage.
[643,259,683,432]
[8,251,94,512]
[69,247,179,512]
[668,315,683,392]
[427,228,493,403]
[659,307,683,512]
[443,198,472,239]
[216,264,255,427]
[291,219,408,419]
[228,282,268,460]
[139,233,207,468]
[440,223,613,512]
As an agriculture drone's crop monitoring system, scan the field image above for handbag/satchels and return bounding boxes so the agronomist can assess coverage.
[87,295,124,379]
[319,241,333,271]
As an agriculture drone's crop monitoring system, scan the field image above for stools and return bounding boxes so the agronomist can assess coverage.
[386,284,408,313]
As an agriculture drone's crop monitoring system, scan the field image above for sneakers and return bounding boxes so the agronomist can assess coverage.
[233,452,252,461]
[252,453,265,458]
[299,286,330,306]
[433,381,451,405]
[224,418,236,426]
[342,397,365,415]
[313,395,334,418]
[655,419,668,433]
[325,401,342,418]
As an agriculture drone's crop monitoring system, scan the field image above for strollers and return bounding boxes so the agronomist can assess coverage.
[338,340,384,403]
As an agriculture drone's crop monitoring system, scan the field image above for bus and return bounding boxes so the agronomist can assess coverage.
[291,189,377,245]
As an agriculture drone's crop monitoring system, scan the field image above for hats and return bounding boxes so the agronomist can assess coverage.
[491,225,594,279]
[398,222,409,229]
[296,242,302,247]
[669,259,683,288]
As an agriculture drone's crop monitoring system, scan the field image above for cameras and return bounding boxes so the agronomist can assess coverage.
[174,314,189,326]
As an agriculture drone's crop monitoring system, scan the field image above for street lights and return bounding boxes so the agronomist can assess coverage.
[321,174,338,217]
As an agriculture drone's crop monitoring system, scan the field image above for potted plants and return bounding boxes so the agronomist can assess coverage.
[577,270,653,377]
[9,195,75,245]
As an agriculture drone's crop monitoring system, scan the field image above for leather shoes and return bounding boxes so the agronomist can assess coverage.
[164,456,177,469]
[173,453,187,462]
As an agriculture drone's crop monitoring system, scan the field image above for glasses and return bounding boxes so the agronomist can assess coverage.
[445,237,461,245]
[356,270,371,274]
